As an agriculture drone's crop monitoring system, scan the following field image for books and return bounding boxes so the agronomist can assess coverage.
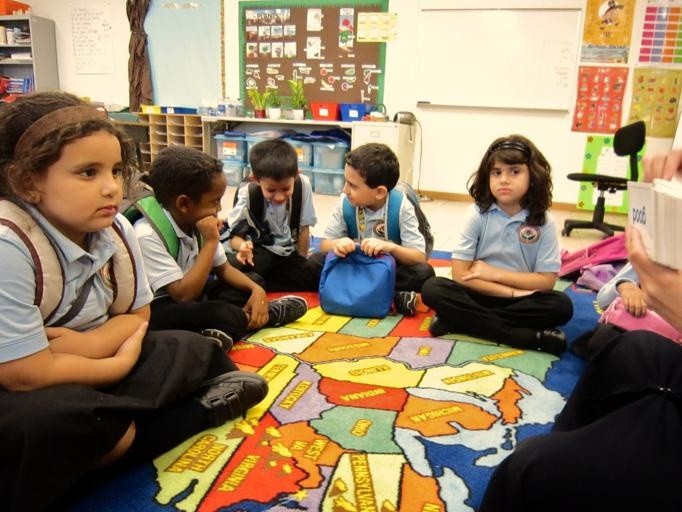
[0,30,34,96]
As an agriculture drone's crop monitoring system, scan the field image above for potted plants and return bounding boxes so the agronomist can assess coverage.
[248,83,266,118]
[286,72,308,119]
[266,91,285,118]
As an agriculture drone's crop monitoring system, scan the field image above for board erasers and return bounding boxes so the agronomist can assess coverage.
[417,102,430,104]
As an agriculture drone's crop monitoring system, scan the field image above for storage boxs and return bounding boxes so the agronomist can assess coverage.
[338,101,367,121]
[213,132,348,196]
[2,0,32,16]
[309,100,341,120]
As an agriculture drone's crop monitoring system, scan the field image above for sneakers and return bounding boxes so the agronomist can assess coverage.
[392,290,417,316]
[264,294,309,329]
[198,328,234,355]
[187,370,270,432]
[429,313,449,337]
[538,327,567,356]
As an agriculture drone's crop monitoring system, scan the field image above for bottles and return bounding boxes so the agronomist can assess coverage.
[216,96,243,117]
[393,111,416,125]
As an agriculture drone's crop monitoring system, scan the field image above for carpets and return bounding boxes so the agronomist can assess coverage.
[66,234,607,512]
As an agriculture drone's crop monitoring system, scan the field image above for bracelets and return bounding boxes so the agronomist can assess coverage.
[512,286,514,298]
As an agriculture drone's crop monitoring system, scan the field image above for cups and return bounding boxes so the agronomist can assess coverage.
[0,26,7,45]
[5,28,16,46]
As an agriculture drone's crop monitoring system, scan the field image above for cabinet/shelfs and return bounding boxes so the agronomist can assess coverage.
[0,12,61,101]
[137,111,203,175]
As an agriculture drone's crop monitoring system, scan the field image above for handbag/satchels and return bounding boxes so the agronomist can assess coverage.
[318,246,399,319]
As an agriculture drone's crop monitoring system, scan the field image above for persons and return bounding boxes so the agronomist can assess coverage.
[421,134,573,354]
[476,147,682,512]
[2,92,270,512]
[298,143,435,315]
[218,138,327,297]
[119,145,308,355]
[573,258,680,359]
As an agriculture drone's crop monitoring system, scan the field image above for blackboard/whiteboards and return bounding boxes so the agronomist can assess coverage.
[416,6,583,113]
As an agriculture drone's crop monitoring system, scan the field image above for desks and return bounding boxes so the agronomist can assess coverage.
[202,115,417,187]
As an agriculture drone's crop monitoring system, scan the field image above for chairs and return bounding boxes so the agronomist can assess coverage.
[561,120,646,241]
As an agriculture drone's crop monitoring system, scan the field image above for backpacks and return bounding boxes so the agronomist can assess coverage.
[342,179,435,261]
[118,187,204,263]
[232,174,303,247]
